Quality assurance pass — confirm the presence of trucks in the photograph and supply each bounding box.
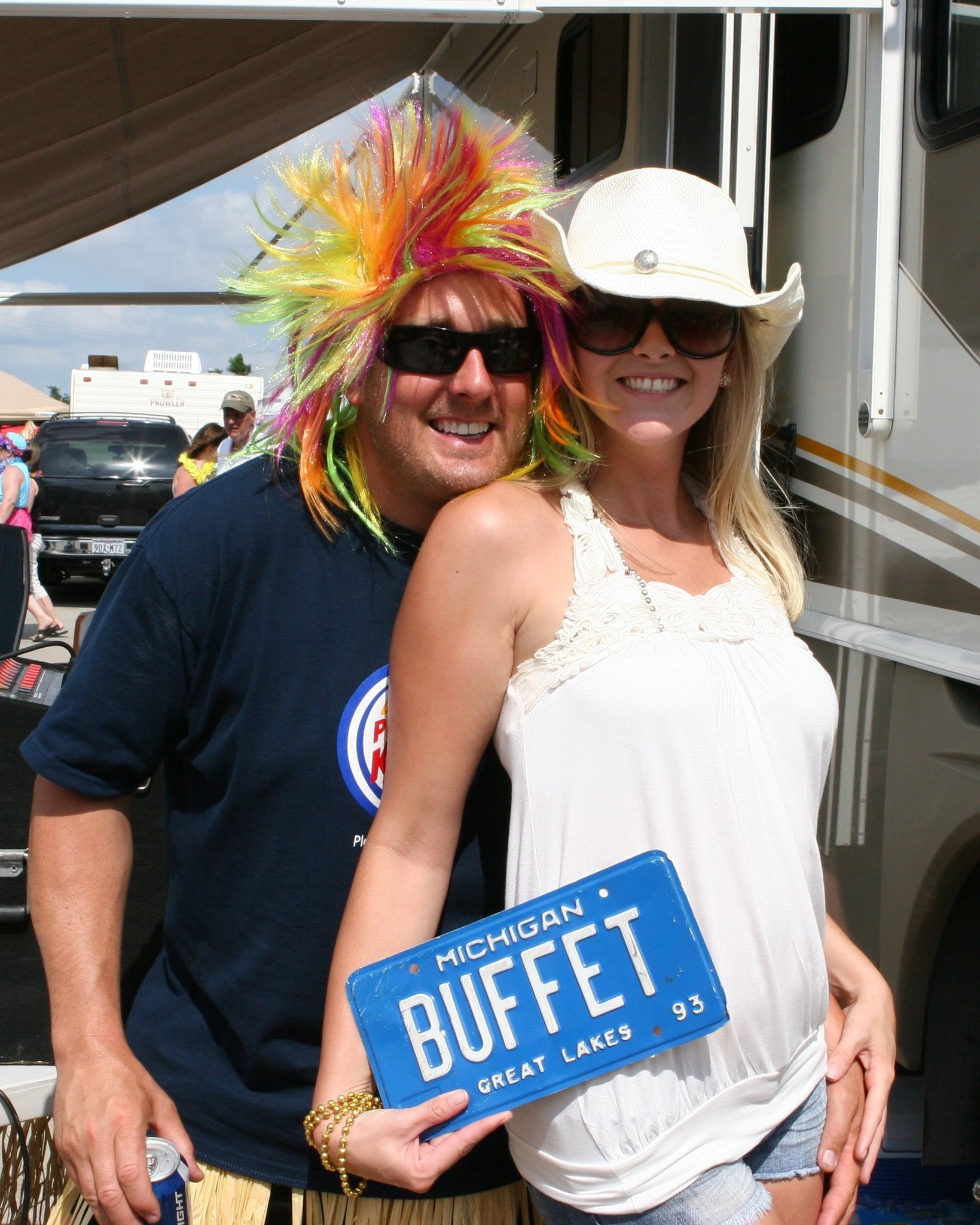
[70,366,263,446]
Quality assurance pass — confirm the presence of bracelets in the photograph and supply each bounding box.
[304,1091,384,1200]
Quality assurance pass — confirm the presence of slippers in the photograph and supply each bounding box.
[32,624,68,642]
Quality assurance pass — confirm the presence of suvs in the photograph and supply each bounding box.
[29,412,193,597]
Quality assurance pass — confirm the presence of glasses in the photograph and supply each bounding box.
[375,323,543,377]
[563,290,743,360]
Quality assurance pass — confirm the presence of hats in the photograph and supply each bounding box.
[218,390,255,413]
[529,166,806,369]
[0,432,27,457]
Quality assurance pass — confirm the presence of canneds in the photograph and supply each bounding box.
[138,1137,190,1225]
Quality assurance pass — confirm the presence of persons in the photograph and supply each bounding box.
[17,105,872,1225]
[312,169,901,1225]
[0,390,275,643]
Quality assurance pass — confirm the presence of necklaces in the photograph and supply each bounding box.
[30,470,43,479]
[179,450,214,484]
[0,456,23,474]
